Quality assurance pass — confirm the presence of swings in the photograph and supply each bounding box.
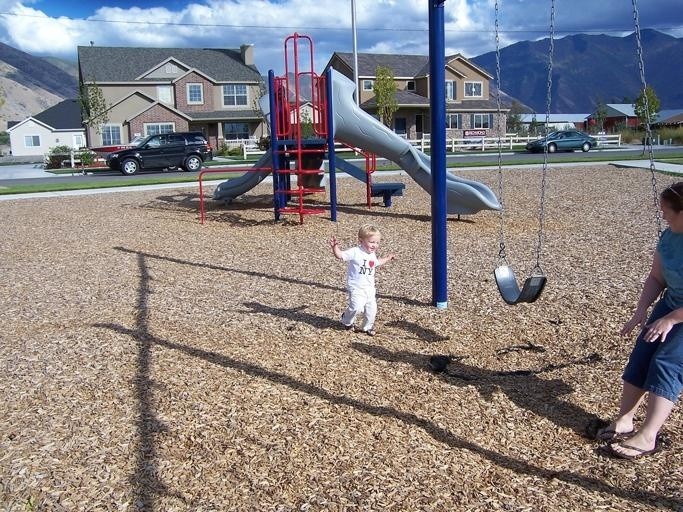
[494,0,555,306]
[630,1,683,309]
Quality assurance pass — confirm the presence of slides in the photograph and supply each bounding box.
[212,151,274,206]
[331,68,503,215]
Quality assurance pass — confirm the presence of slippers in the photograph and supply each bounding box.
[610,439,662,460]
[595,424,636,445]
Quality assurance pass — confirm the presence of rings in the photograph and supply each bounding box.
[658,331,661,335]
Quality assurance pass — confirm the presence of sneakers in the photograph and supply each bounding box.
[344,323,360,333]
[362,329,377,337]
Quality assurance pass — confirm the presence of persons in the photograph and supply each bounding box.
[596,182,683,459]
[330,224,396,335]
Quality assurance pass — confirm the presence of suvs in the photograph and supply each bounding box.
[105,131,213,177]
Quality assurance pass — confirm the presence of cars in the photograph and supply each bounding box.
[525,130,593,154]
